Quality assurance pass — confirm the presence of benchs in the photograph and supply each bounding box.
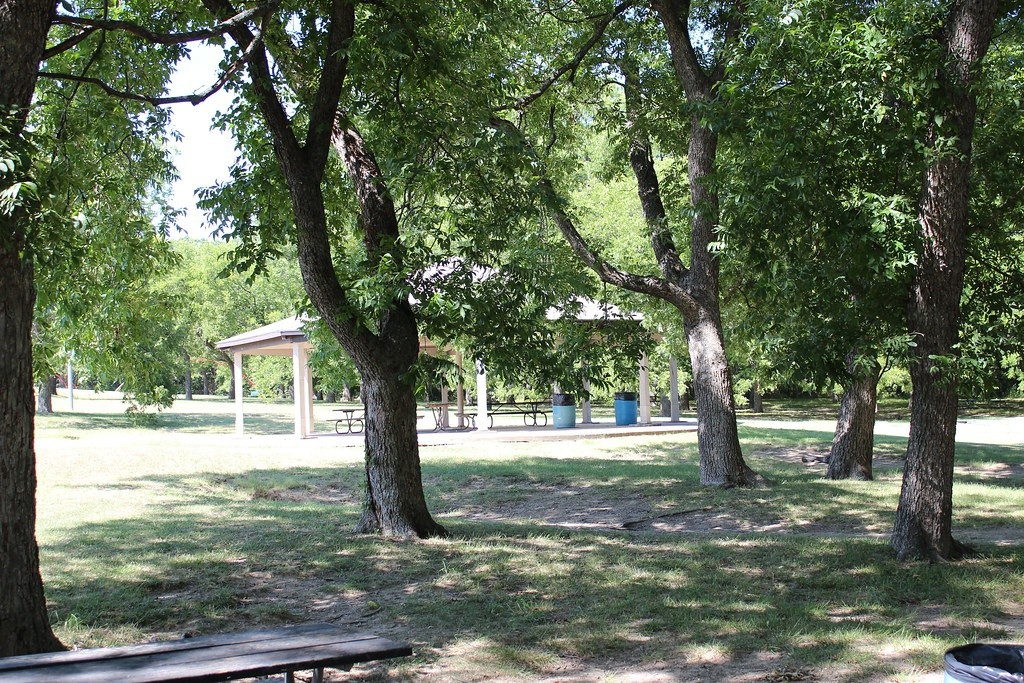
[454,410,554,432]
[327,417,365,422]
[0,624,413,683]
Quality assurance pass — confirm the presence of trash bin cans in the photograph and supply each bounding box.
[943,642,1024,683]
[553,394,576,428]
[615,392,637,426]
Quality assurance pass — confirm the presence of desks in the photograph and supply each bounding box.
[487,401,552,428]
[426,403,449,432]
[332,408,364,434]
